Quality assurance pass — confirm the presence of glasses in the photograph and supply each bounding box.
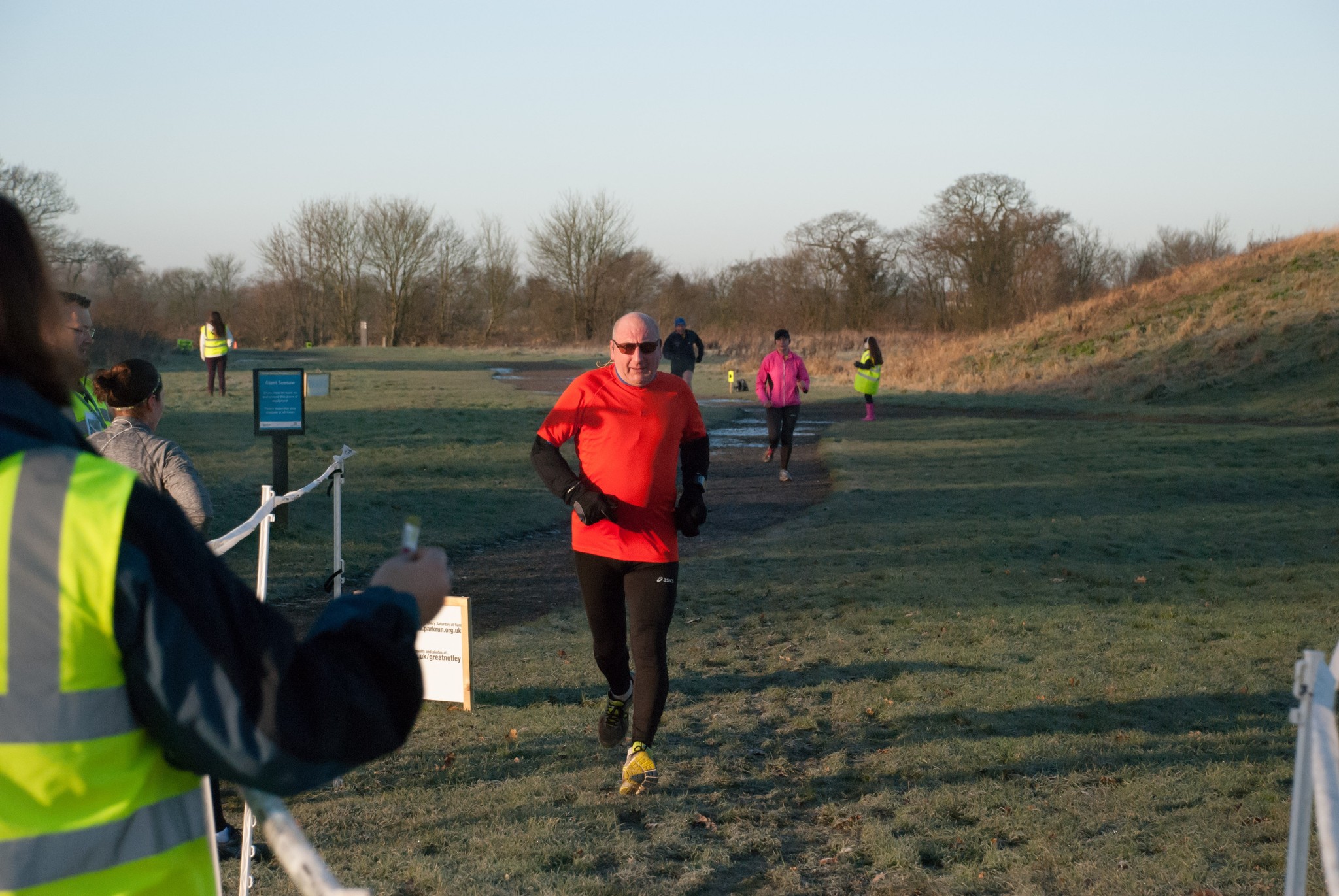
[611,339,658,355]
[58,324,96,339]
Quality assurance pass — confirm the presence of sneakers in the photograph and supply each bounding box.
[618,742,658,797]
[597,671,638,747]
[779,469,792,482]
[763,446,775,463]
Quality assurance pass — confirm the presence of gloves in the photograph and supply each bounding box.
[678,487,707,527]
[565,485,617,526]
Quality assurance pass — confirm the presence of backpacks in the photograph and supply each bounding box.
[735,379,749,392]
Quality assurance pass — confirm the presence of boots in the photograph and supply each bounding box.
[864,403,875,422]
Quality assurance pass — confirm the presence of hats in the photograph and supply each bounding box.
[674,318,686,328]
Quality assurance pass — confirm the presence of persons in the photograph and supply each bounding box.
[0,194,450,896]
[199,310,235,397]
[660,318,703,393]
[43,288,117,438]
[755,329,810,482]
[78,358,214,541]
[531,310,711,798]
[853,335,884,421]
